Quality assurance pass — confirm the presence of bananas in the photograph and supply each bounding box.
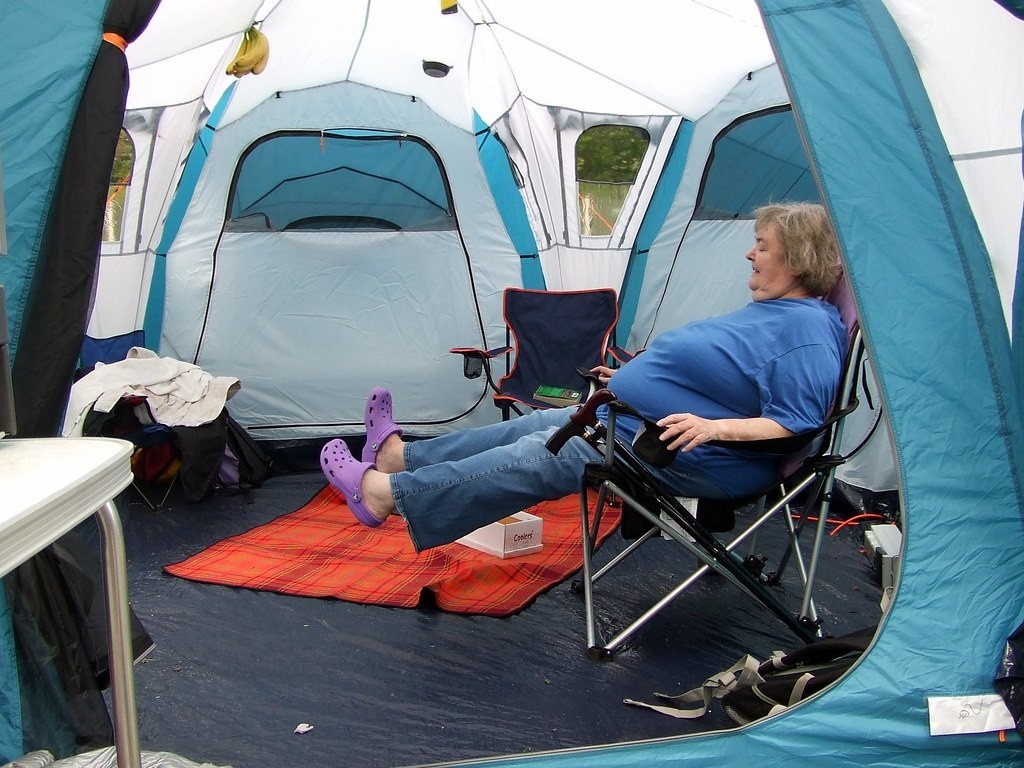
[225,26,269,78]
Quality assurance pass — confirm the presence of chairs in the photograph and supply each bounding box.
[81,331,179,511]
[450,286,632,422]
[545,267,886,664]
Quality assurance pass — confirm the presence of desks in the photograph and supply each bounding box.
[0,436,143,768]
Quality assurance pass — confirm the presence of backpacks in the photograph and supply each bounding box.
[623,624,879,727]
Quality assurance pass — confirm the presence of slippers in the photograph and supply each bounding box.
[361,389,403,464]
[320,439,387,527]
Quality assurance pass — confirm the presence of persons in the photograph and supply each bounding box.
[320,201,848,554]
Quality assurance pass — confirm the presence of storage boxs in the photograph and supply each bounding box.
[454,511,542,558]
